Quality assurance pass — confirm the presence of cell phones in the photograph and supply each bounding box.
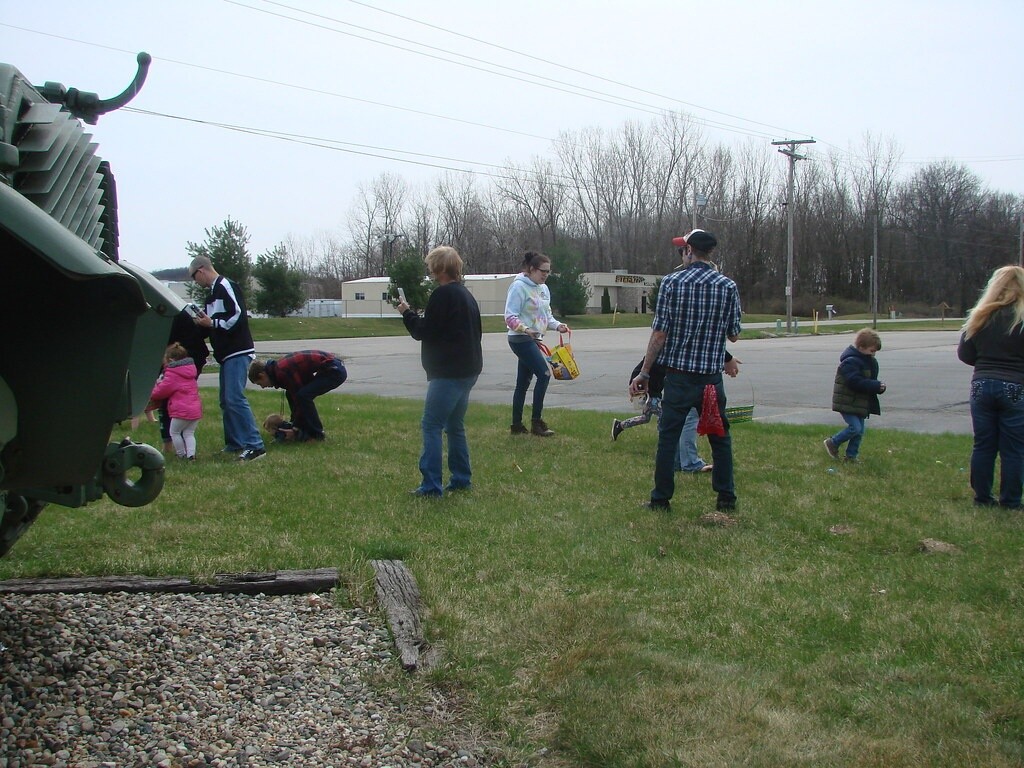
[398,287,409,305]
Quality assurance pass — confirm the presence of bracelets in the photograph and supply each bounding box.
[640,372,650,378]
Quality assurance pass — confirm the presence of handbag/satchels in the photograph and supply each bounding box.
[539,328,580,381]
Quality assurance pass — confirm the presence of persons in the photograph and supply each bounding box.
[504,251,568,437]
[398,246,484,497]
[957,265,1024,512]
[629,229,741,513]
[823,329,886,465]
[159,309,210,453]
[188,256,266,464]
[612,355,666,442]
[151,342,202,461]
[674,406,713,474]
[249,349,347,443]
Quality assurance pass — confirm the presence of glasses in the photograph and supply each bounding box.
[190,266,202,281]
[536,268,552,276]
[678,246,689,255]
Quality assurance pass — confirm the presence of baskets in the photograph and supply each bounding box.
[723,370,756,423]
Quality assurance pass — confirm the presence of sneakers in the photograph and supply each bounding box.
[511,422,532,433]
[531,420,555,436]
[237,449,265,461]
[221,445,237,452]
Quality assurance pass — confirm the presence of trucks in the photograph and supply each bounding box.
[0,46,181,559]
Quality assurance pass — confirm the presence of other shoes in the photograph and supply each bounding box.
[176,454,187,462]
[824,438,839,458]
[842,456,861,464]
[188,455,196,462]
[445,484,470,493]
[408,489,423,496]
[611,418,624,441]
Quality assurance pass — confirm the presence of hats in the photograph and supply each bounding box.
[688,232,717,252]
[672,228,705,247]
